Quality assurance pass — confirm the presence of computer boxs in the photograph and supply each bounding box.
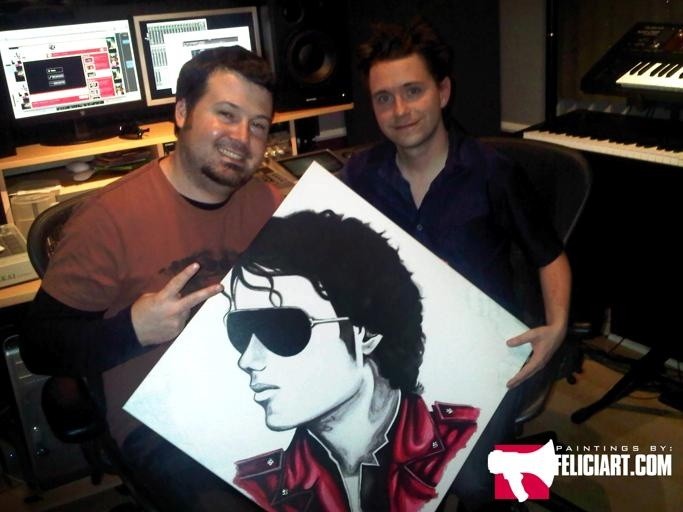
[3,335,106,489]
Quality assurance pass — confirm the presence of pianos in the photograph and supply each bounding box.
[581,21,683,102]
[512,109,683,168]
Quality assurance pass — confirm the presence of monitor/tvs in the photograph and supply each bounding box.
[132,6,264,109]
[0,16,148,147]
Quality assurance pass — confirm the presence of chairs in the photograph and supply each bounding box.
[26,181,186,510]
[466,128,598,511]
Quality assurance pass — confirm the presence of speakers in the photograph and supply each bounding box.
[257,0,353,108]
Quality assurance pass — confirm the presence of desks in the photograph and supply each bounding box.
[0,95,393,311]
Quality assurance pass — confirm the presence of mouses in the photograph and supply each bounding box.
[66,161,90,173]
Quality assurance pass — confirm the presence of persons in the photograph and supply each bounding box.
[218,208,482,512]
[344,14,575,512]
[19,45,287,511]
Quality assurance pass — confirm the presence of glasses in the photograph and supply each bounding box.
[226,307,349,357]
